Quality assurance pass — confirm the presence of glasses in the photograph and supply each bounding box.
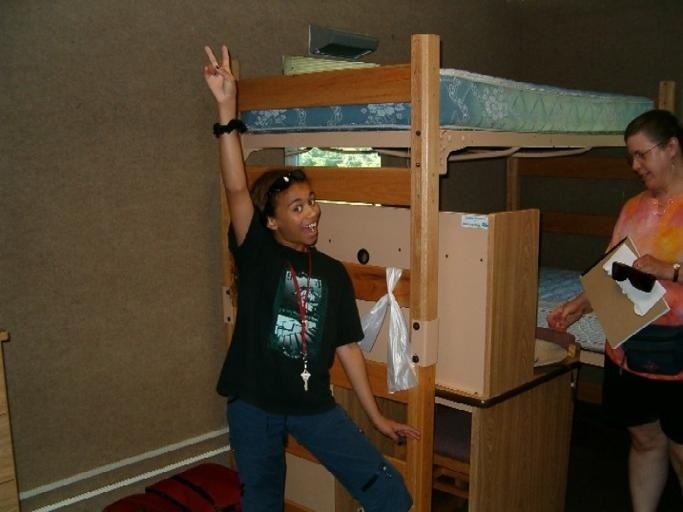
[623,128,682,170]
[610,259,657,294]
[267,168,305,194]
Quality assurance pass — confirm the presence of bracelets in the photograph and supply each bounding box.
[212,119,248,138]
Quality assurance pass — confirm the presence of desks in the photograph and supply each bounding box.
[313,203,583,512]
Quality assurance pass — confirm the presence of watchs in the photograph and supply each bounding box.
[672,263,681,282]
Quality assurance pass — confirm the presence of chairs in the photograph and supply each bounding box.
[453,328,577,511]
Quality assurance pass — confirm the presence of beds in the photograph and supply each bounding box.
[214,33,678,512]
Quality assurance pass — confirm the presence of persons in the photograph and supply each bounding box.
[547,109,682,512]
[202,45,422,512]
